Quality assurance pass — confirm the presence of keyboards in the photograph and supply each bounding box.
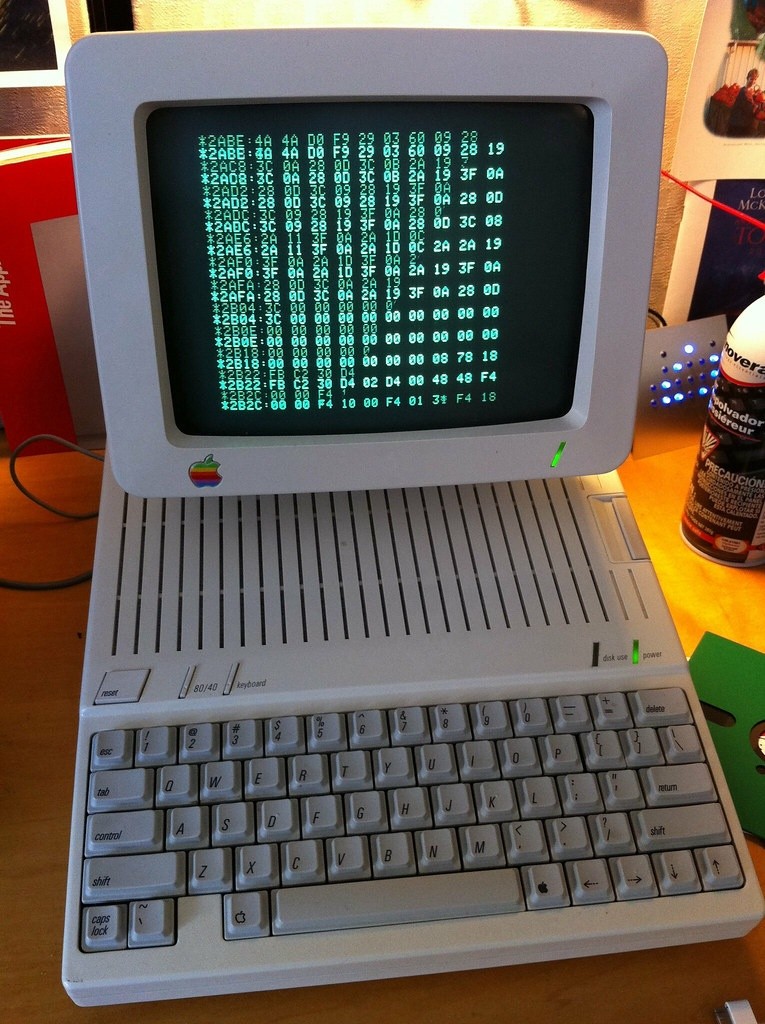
[77,687,746,952]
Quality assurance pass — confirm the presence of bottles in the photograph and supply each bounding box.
[680,296,765,570]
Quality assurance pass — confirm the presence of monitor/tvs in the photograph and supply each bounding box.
[0,0,73,88]
[72,26,669,498]
